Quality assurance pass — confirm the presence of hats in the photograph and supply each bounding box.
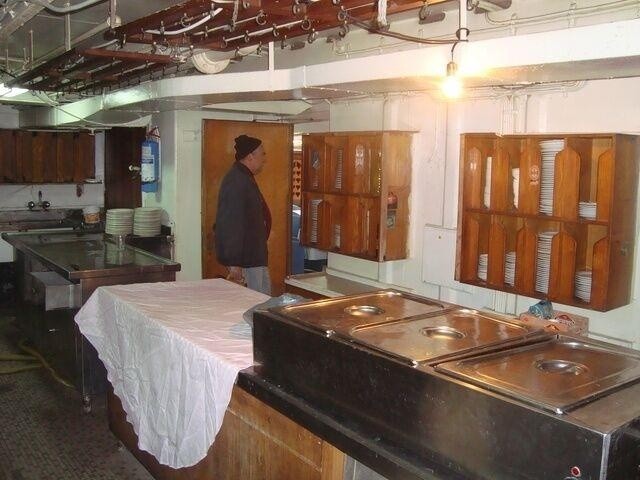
[232,132,263,162]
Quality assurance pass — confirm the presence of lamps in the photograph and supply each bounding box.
[439,60,465,101]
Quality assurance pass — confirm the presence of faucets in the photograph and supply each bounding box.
[39,190,42,203]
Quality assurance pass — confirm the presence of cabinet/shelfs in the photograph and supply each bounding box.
[299,130,421,264]
[453,133,640,312]
[0,127,96,184]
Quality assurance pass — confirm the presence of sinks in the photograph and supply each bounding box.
[16,223,74,231]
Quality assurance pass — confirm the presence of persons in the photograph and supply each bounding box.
[213,133,274,297]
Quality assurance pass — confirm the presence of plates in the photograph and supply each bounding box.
[309,147,345,248]
[105,208,163,236]
[477,141,597,303]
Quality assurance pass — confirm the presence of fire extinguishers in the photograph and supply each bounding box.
[141,126,161,193]
